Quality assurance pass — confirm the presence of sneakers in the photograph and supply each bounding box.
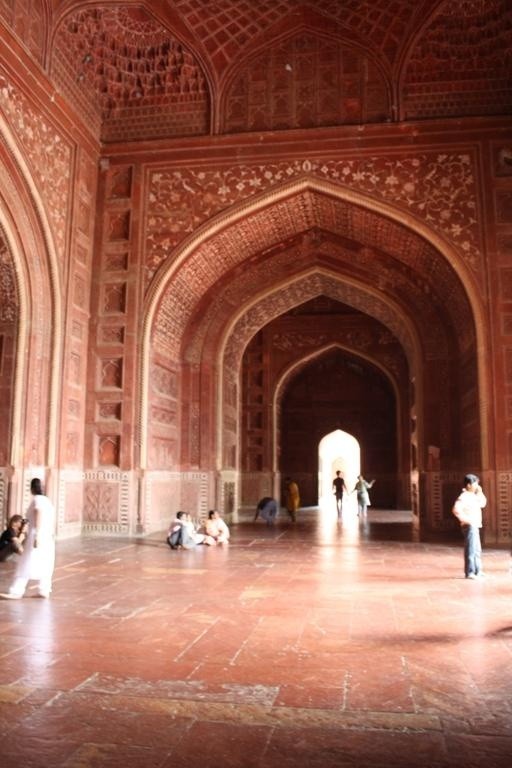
[0,592,22,599]
[467,572,488,579]
[38,592,50,599]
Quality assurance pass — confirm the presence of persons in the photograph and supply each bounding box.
[330,470,377,519]
[251,476,301,528]
[0,478,57,600]
[166,510,231,551]
[451,471,488,578]
